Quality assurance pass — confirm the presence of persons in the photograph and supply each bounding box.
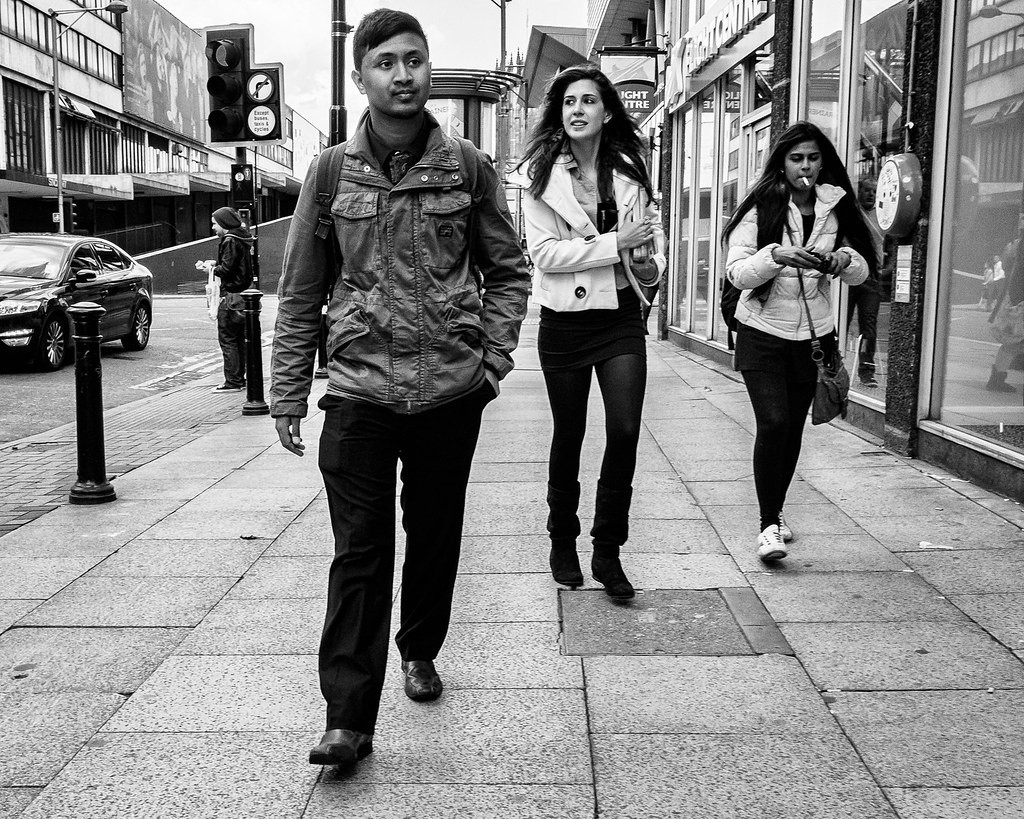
[724,121,877,559]
[270,9,529,764]
[203,207,254,392]
[127,8,205,138]
[514,65,665,600]
[979,230,1024,392]
[847,179,896,386]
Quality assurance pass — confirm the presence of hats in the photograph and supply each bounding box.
[213,207,242,231]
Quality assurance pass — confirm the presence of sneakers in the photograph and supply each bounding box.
[757,524,788,559]
[778,511,794,541]
[859,371,878,387]
[211,382,246,393]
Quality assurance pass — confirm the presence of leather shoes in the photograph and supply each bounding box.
[309,725,374,764]
[402,652,442,701]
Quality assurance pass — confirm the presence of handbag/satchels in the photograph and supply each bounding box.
[990,292,1024,346]
[812,364,849,425]
[206,269,219,321]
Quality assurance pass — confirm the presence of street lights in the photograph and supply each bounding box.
[48,2,129,231]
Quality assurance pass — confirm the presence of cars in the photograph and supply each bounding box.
[0,233,154,372]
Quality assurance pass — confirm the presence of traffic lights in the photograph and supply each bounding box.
[204,24,254,147]
[63,198,77,232]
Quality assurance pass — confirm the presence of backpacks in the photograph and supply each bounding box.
[721,199,846,348]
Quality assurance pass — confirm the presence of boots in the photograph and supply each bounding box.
[986,366,1015,391]
[591,479,635,599]
[547,480,584,586]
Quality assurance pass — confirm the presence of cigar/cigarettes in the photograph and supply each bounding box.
[802,177,811,188]
